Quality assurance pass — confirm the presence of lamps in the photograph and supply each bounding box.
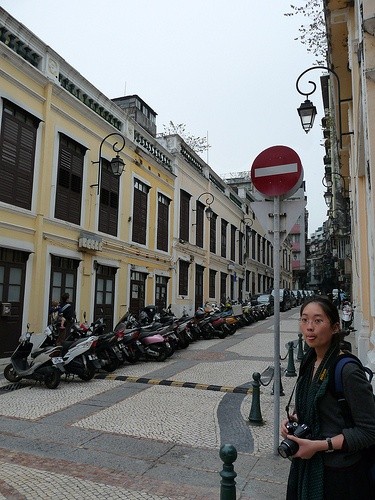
[236,218,253,242]
[295,66,354,148]
[90,133,126,195]
[192,192,214,226]
[321,171,352,235]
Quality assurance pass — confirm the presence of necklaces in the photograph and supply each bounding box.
[314,362,319,368]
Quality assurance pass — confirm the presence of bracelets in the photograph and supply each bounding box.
[325,438,334,453]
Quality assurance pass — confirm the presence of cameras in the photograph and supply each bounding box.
[277,421,312,458]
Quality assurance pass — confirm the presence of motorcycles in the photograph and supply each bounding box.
[27,287,317,391]
[3,321,66,390]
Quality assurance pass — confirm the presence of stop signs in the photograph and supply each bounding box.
[251,145,302,196]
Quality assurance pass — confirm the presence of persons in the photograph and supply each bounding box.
[49,292,76,337]
[281,294,375,500]
